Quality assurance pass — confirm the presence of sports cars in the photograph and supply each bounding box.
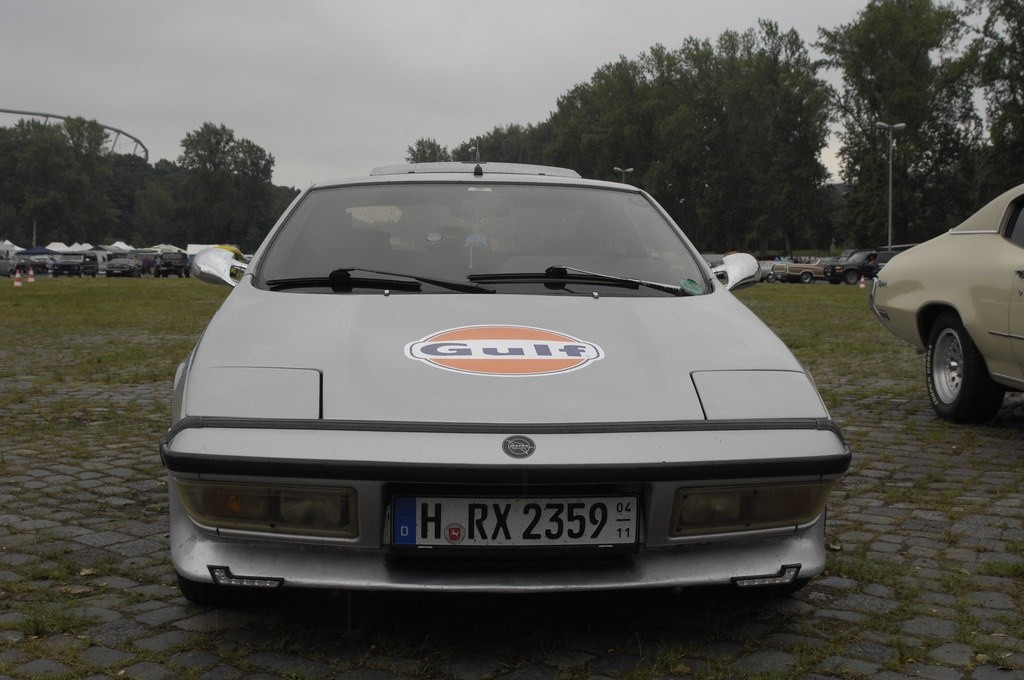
[871,182,1023,425]
[159,161,854,631]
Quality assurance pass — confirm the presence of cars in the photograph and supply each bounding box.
[694,242,926,288]
[0,238,258,279]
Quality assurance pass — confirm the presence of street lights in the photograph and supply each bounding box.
[875,122,908,254]
[612,165,636,185]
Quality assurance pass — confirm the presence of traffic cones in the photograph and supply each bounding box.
[13,268,23,288]
[27,265,36,283]
[858,274,867,287]
